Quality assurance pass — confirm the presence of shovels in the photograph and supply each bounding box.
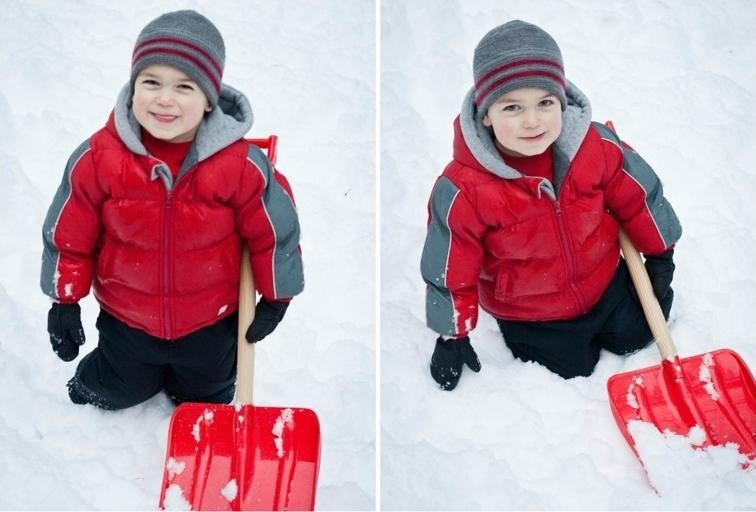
[606,121,756,498]
[160,136,322,511]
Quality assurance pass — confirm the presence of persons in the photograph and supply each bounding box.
[417,16,686,395]
[36,8,307,409]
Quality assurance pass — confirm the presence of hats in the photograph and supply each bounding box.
[130,10,226,110]
[473,20,568,120]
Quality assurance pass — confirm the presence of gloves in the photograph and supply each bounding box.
[228,294,292,344]
[429,336,481,391]
[642,252,678,304]
[45,298,87,364]
